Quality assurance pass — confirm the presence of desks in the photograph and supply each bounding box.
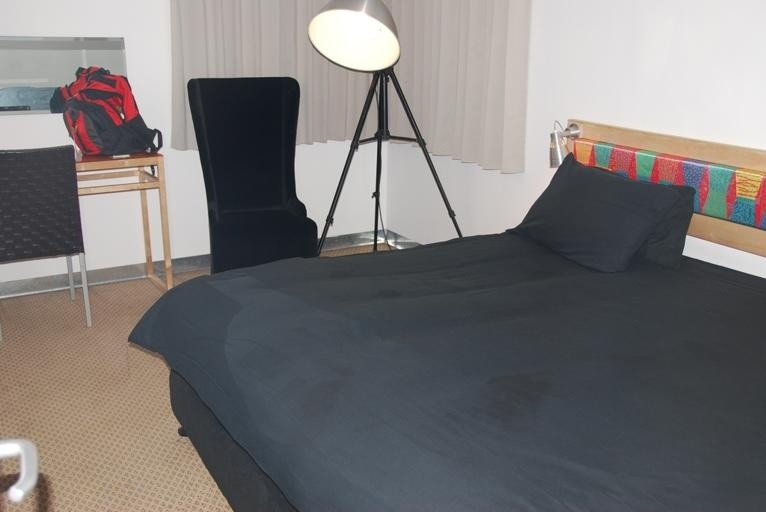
[76,152,173,293]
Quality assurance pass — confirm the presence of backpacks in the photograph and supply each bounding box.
[49,66,163,154]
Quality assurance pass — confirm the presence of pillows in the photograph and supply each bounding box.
[505,151,680,273]
[636,185,695,272]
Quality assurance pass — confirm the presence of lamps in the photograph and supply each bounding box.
[308,0,464,255]
[549,120,580,168]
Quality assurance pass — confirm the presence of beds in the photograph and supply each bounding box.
[129,119,766,512]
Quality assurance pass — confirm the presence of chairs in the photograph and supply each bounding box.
[186,76,319,277]
[0,145,92,343]
